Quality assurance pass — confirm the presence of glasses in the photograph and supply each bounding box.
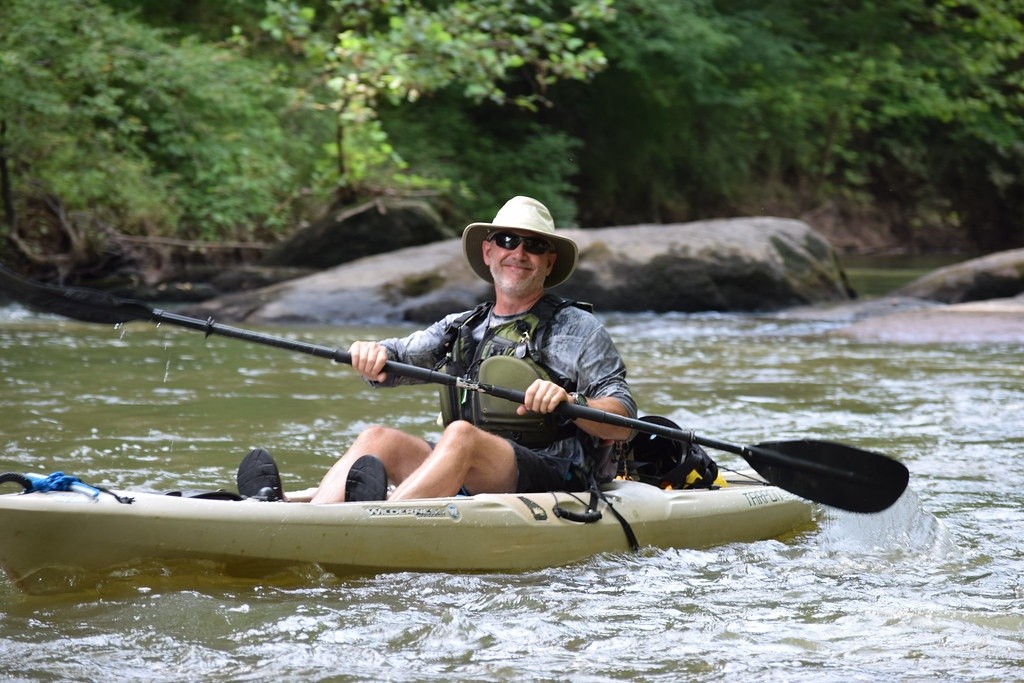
[488,232,553,255]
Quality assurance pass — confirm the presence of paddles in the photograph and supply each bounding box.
[29,267,909,515]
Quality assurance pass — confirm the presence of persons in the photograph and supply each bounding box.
[235,195,637,504]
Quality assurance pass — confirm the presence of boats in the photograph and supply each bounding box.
[1,470,827,596]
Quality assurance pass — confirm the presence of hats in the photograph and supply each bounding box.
[461,196,579,291]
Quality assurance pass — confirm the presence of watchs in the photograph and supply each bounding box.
[567,391,588,405]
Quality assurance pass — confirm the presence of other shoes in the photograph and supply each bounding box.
[237,450,284,503]
[344,455,387,502]
